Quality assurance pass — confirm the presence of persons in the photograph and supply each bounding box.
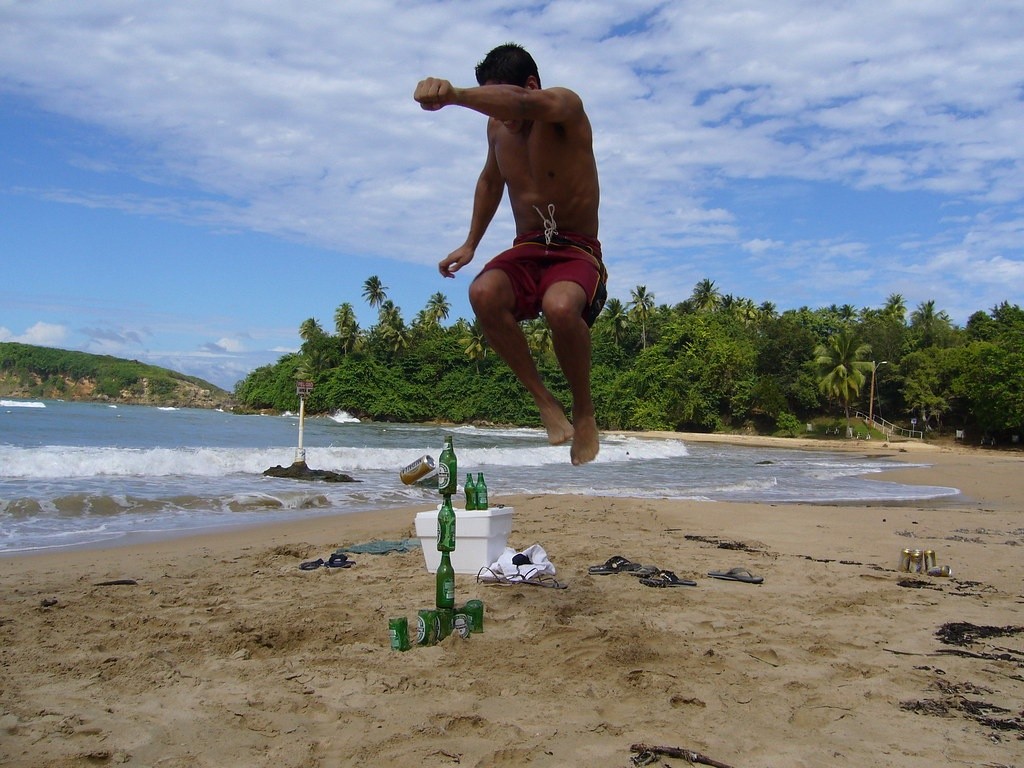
[413,40,609,466]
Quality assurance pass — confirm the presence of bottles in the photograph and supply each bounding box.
[435,551,454,607]
[436,494,456,551]
[475,472,488,511]
[464,472,477,510]
[437,435,457,494]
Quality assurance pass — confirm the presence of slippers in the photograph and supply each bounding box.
[515,567,568,590]
[629,564,660,578]
[589,556,642,575]
[639,568,699,587]
[707,565,764,585]
[325,553,355,568]
[297,558,323,570]
[476,566,510,589]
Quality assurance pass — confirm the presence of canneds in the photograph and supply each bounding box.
[400,455,436,486]
[388,599,483,651]
[897,546,952,577]
